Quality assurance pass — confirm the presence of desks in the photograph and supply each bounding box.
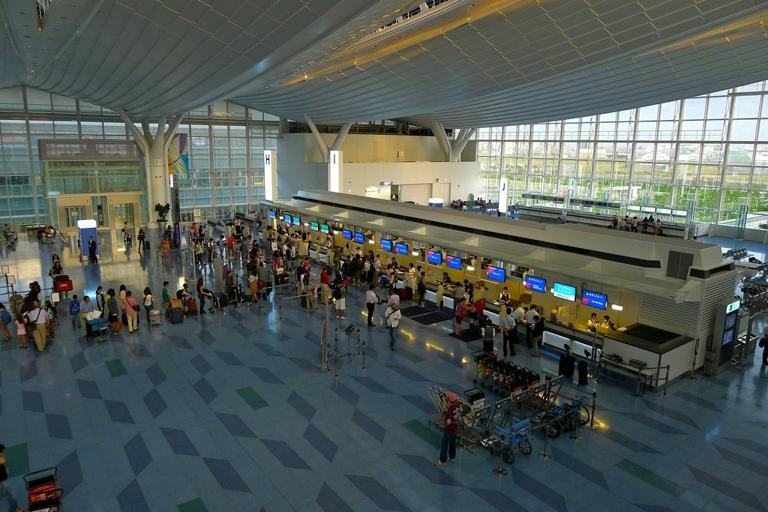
[600,356,653,396]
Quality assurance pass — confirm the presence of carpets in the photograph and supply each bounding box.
[399,304,482,343]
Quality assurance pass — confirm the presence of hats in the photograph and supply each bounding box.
[447,391,457,402]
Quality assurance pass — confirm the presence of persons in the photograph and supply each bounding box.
[453,197,491,211]
[602,314,615,331]
[588,312,600,333]
[610,215,662,236]
[762,326,768,365]
[434,393,463,465]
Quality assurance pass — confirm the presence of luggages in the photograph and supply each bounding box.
[301,289,312,308]
[53,274,73,292]
[149,309,160,326]
[162,298,198,324]
[122,311,129,326]
[204,283,246,313]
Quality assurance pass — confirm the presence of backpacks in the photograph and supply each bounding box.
[333,285,343,301]
[176,290,183,300]
[49,307,57,320]
[0,308,11,325]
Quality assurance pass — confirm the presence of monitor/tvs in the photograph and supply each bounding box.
[525,275,546,293]
[581,289,608,310]
[553,282,576,302]
[269,207,462,271]
[487,266,506,283]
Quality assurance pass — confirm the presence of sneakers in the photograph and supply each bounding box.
[435,460,447,466]
[336,315,346,319]
[448,457,454,462]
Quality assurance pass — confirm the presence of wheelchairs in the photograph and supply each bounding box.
[483,394,590,464]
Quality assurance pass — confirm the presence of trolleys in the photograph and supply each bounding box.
[205,283,253,315]
[83,311,110,343]
[425,349,563,443]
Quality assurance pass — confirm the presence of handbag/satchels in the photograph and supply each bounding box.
[114,321,122,331]
[71,304,79,315]
[24,322,36,332]
[133,304,141,312]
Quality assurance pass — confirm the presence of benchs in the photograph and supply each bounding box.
[2,224,61,247]
